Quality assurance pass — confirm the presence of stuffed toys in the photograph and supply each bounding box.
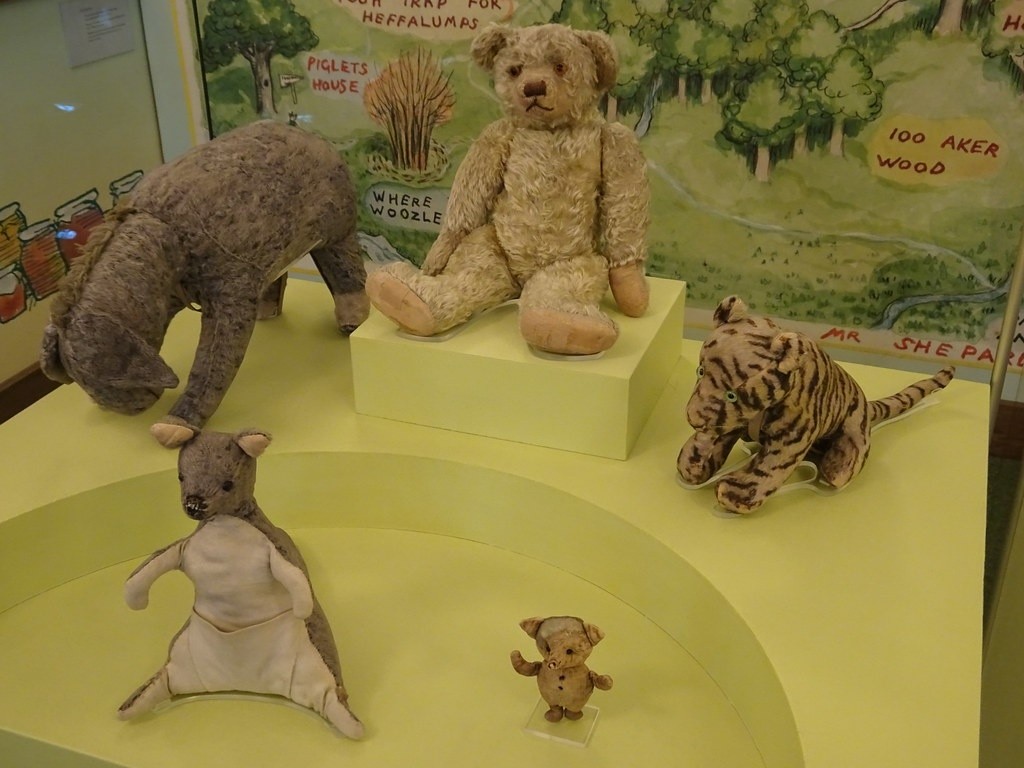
[678,295,953,513]
[513,616,614,721]
[114,422,367,742]
[366,24,649,355]
[42,124,370,446]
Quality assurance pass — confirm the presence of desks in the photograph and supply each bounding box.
[0,280,988,768]
